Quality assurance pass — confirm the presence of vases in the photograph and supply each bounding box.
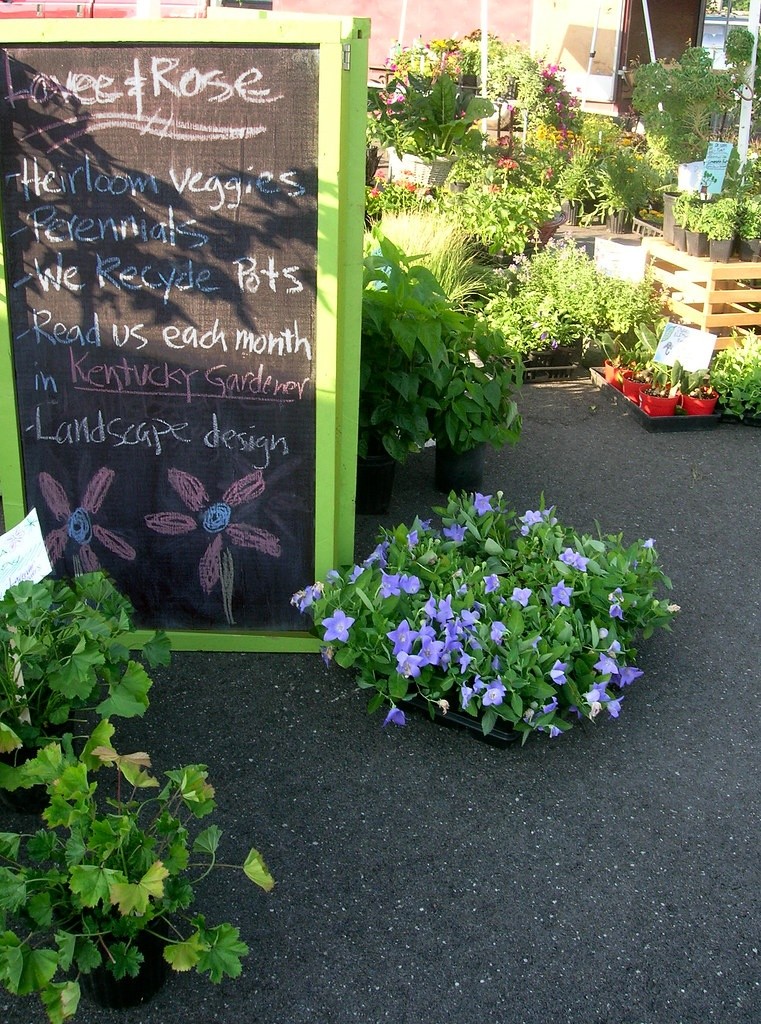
[392,674,523,747]
[528,351,554,379]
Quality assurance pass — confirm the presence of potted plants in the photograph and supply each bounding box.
[583,308,761,426]
[362,74,761,264]
[0,577,275,1024]
[356,225,526,512]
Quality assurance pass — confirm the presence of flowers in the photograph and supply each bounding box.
[527,327,560,353]
[293,486,685,741]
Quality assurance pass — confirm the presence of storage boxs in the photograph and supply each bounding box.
[590,367,721,434]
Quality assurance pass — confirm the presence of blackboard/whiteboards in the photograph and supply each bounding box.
[0,16,372,653]
